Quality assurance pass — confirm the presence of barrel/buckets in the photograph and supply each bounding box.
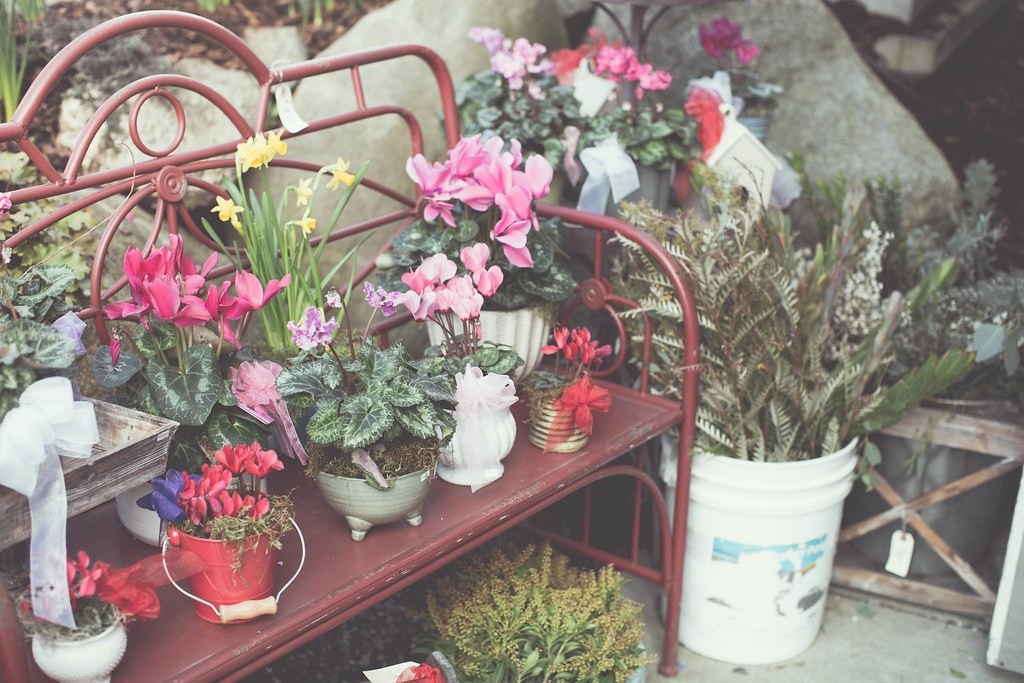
[650,396,865,667]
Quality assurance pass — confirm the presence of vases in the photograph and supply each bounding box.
[313,468,433,542]
[29,618,128,683]
[734,115,770,145]
[162,517,306,625]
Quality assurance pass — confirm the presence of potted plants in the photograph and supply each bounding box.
[614,154,1023,666]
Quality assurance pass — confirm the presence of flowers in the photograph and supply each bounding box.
[697,14,785,120]
[0,114,636,493]
[141,431,297,590]
[10,544,164,641]
[0,7,703,683]
